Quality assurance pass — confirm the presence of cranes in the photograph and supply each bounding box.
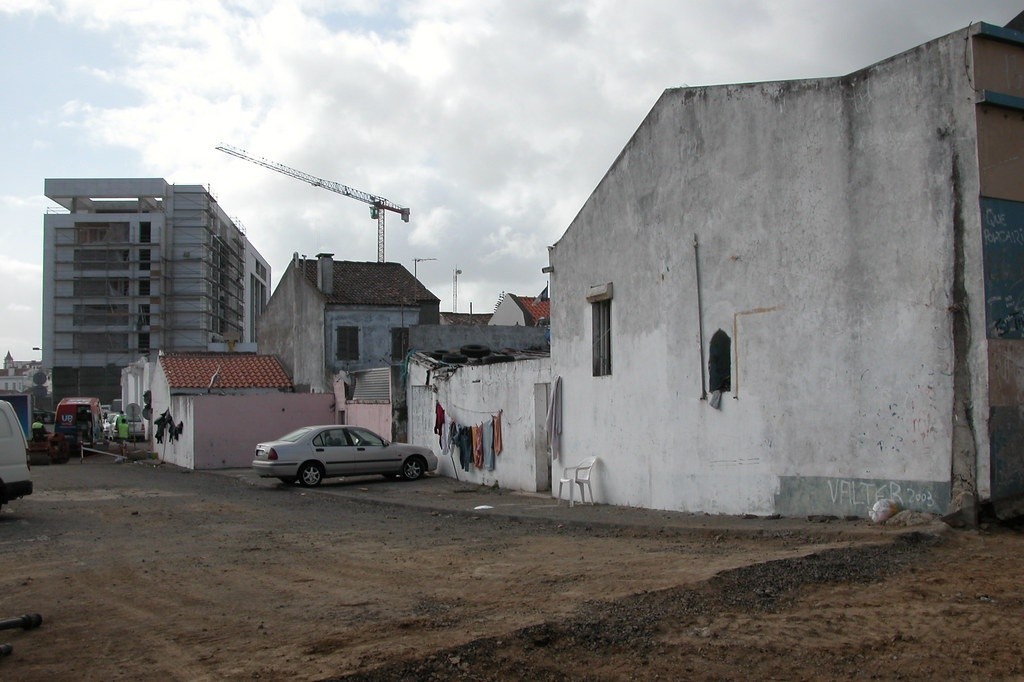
[211,140,410,262]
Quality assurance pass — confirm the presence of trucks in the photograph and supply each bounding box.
[53,396,100,447]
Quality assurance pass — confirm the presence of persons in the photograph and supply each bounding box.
[117,419,128,443]
[32,417,51,442]
[115,411,126,430]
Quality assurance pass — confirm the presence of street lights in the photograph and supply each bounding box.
[31,346,85,397]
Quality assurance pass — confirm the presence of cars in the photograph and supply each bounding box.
[0,398,35,510]
[252,426,439,489]
[100,399,145,440]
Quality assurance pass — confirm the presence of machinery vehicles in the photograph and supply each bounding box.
[451,265,462,312]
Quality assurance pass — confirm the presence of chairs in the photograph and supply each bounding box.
[558,455,598,508]
